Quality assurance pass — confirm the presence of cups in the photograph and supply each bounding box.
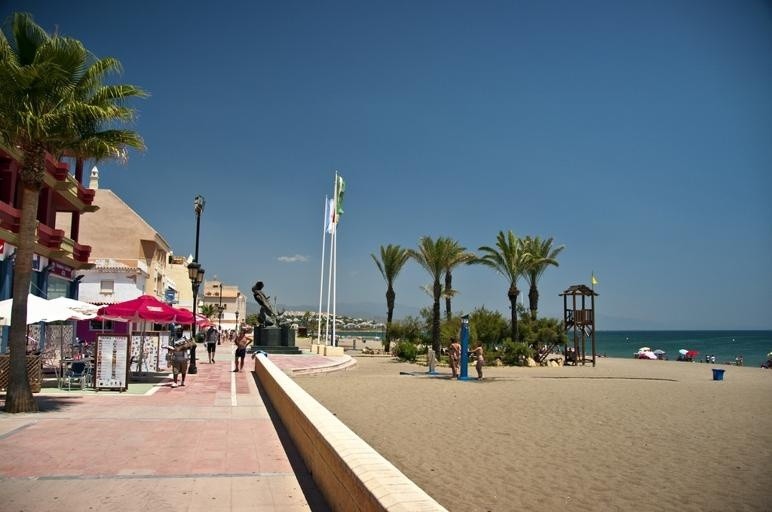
[81,353,85,359]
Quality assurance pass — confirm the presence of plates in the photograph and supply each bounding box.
[64,358,75,361]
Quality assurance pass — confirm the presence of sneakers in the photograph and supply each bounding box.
[171,381,184,387]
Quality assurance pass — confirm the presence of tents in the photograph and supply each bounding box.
[639,352,657,359]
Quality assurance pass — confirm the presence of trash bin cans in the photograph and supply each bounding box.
[197,333,205,343]
[712,369,725,380]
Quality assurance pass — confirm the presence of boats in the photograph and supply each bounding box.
[633,347,654,356]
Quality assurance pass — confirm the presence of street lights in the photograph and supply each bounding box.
[461,315,468,377]
[217,284,225,344]
[233,311,239,333]
[187,194,205,374]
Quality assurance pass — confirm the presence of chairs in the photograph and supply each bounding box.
[129,353,149,375]
[58,362,85,392]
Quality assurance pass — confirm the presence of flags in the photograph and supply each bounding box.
[325,174,346,234]
[592,277,599,284]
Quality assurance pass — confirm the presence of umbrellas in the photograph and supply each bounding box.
[687,350,697,356]
[654,349,665,354]
[639,346,650,351]
[679,348,688,355]
[0,294,216,389]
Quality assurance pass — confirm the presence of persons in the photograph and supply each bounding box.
[470,340,484,378]
[232,330,253,372]
[735,356,739,365]
[678,353,695,362]
[740,356,743,366]
[700,354,715,363]
[448,336,461,378]
[221,328,236,343]
